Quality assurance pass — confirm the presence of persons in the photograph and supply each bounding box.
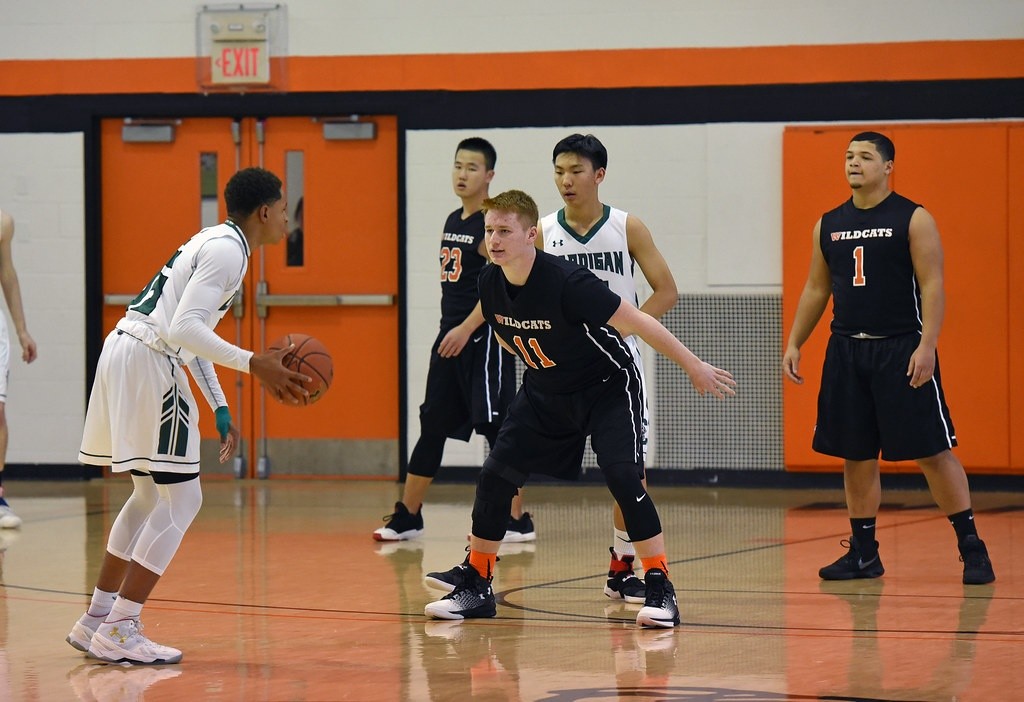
[374,539,678,702]
[780,132,997,585]
[421,191,738,630]
[1,211,37,529]
[67,663,184,702]
[819,580,996,702]
[371,135,537,544]
[286,197,303,266]
[424,130,680,602]
[61,167,312,666]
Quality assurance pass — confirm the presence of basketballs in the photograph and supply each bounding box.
[265,332,336,407]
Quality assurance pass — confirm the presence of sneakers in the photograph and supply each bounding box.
[87,618,183,665]
[958,584,995,624]
[637,625,682,669]
[957,535,996,586]
[425,552,470,591]
[425,562,497,620]
[0,487,23,529]
[636,567,680,628]
[67,659,184,700]
[372,501,425,542]
[496,543,536,571]
[820,578,885,614]
[373,540,424,572]
[65,609,109,653]
[604,601,645,630]
[424,617,497,670]
[604,547,647,605]
[467,511,537,542]
[818,536,885,580]
